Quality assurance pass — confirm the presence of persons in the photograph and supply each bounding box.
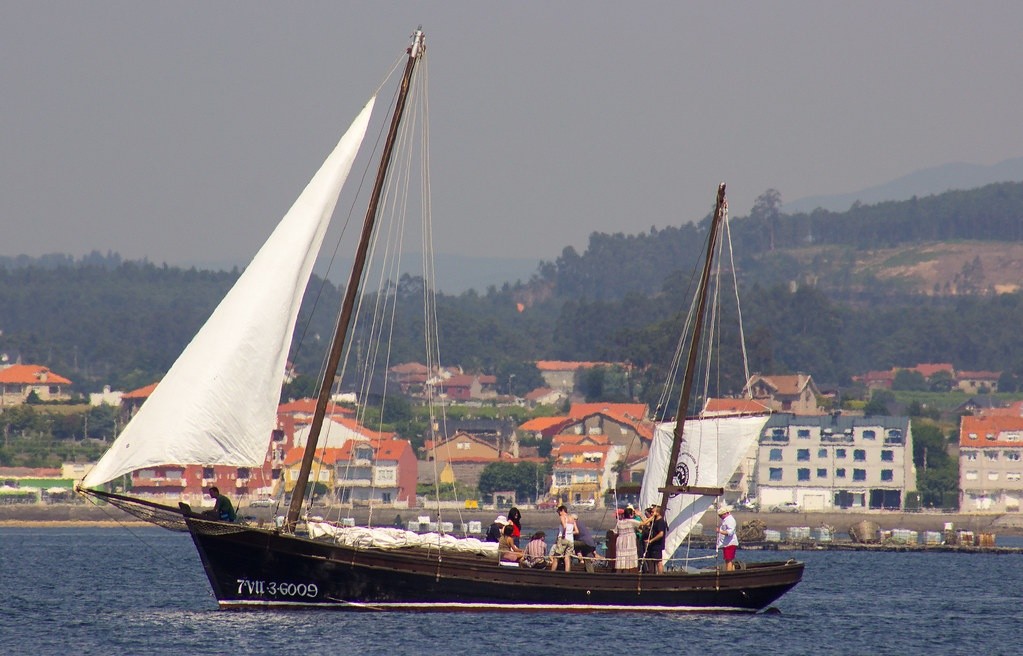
[610,506,665,574]
[486,506,601,572]
[714,507,739,571]
[201,487,235,523]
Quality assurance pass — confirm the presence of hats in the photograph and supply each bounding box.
[535,532,548,539]
[611,509,624,517]
[718,508,729,514]
[494,516,509,526]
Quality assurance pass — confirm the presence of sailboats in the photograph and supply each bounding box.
[74,22,808,616]
